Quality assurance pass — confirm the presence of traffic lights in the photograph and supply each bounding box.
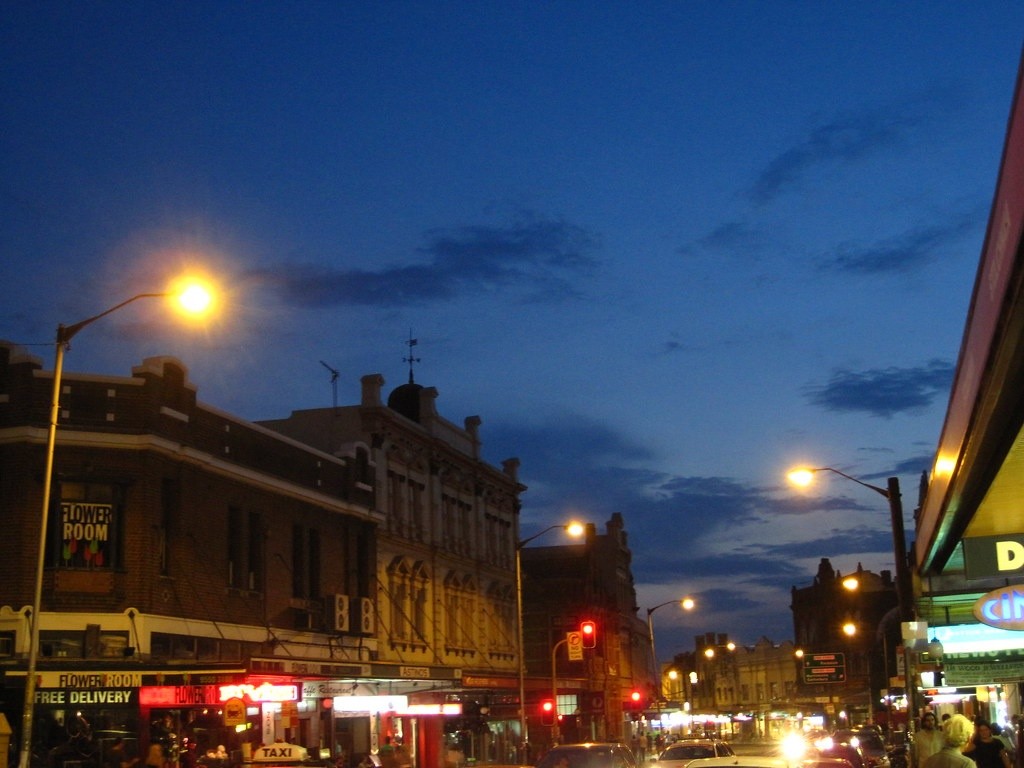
[539,700,555,727]
[631,688,643,715]
[581,621,597,650]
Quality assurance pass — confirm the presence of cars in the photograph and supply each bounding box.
[655,737,738,768]
[791,722,892,768]
[534,742,636,768]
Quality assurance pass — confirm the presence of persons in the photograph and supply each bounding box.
[380,736,395,768]
[865,718,872,725]
[557,732,618,745]
[358,756,375,768]
[525,743,531,765]
[630,727,675,762]
[888,721,907,745]
[336,758,344,768]
[914,711,1024,768]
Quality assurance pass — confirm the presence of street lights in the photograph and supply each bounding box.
[842,622,894,739]
[782,465,915,730]
[513,520,586,768]
[647,596,697,747]
[793,648,851,726]
[18,276,222,767]
